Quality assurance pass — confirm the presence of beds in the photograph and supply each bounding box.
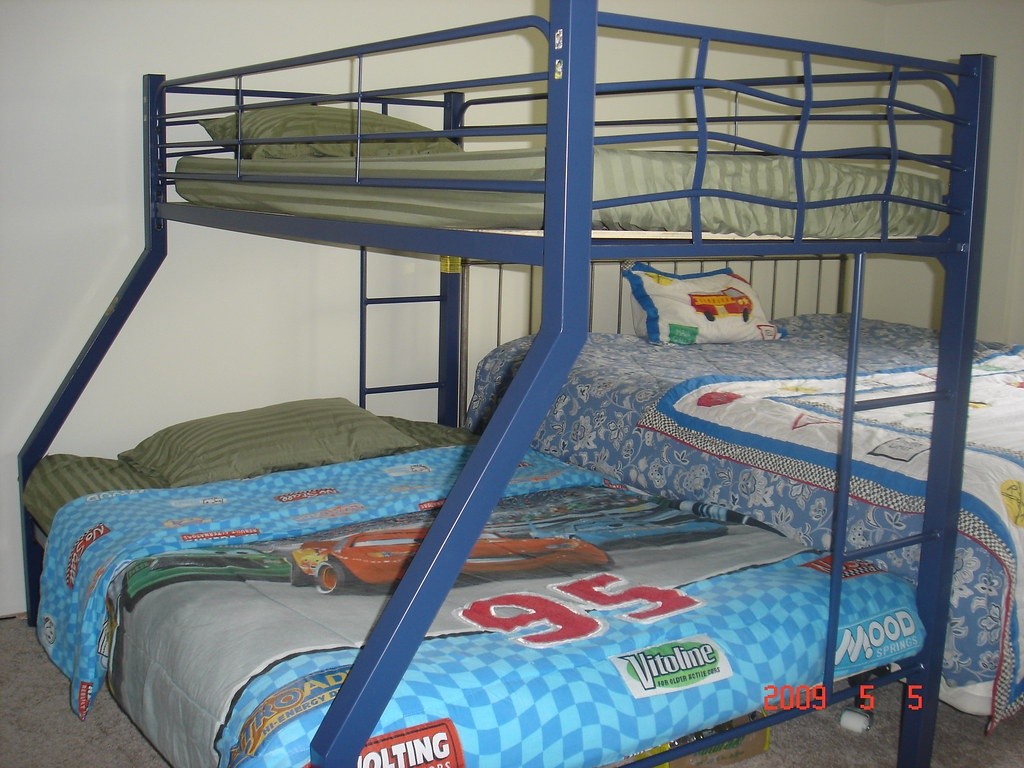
[459,258,1023,735]
[19,1,998,768]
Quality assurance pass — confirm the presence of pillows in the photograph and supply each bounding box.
[117,397,417,487]
[624,262,787,346]
[199,105,463,161]
